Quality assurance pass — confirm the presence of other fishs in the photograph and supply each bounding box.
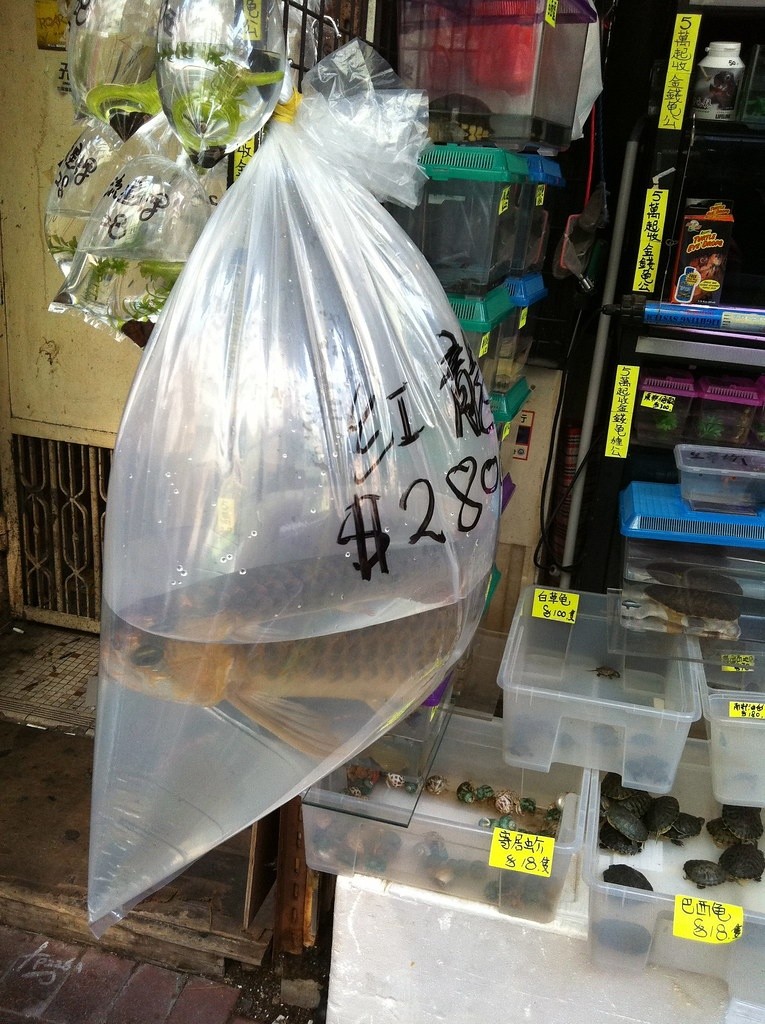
[98,487,494,765]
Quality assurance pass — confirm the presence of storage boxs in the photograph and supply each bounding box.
[298,1,765,1024]
